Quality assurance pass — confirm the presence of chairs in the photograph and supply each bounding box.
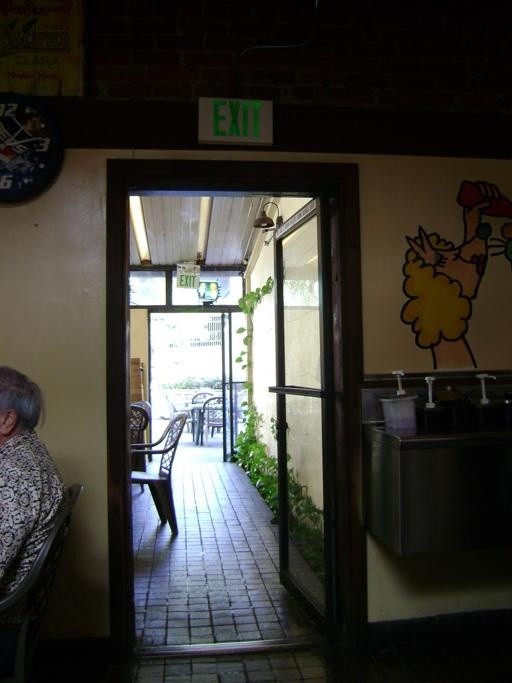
[132,391,227,538]
[0,483,85,683]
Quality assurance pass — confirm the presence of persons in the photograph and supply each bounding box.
[0,366,69,625]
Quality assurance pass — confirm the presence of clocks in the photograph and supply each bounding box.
[0,91,69,210]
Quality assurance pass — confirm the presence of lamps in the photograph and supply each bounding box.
[198,278,220,305]
[252,199,283,244]
